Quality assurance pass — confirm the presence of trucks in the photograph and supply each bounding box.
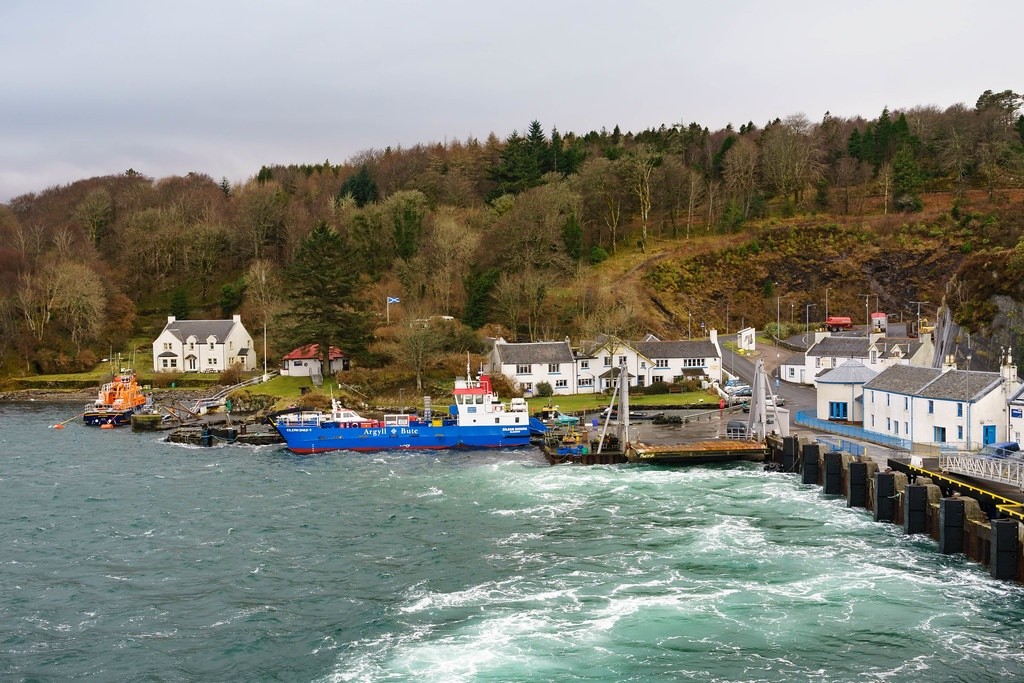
[820,317,853,332]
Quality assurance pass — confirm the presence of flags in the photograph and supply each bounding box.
[389,297,400,303]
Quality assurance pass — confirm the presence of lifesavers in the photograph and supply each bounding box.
[352,422,359,428]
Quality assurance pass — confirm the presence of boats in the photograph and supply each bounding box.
[601,406,665,419]
[267,350,531,454]
[194,402,226,416]
[529,393,584,425]
[47,344,161,428]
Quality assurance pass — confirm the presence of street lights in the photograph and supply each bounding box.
[909,301,930,338]
[778,297,780,340]
[807,304,817,347]
[792,302,794,324]
[826,288,833,321]
[858,294,869,338]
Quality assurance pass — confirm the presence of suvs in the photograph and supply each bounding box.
[973,441,1020,463]
[726,420,770,438]
[729,387,752,396]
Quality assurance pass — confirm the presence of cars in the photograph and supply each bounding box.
[766,394,785,407]
[1001,450,1023,464]
[724,380,750,393]
[760,414,774,423]
[743,398,757,412]
